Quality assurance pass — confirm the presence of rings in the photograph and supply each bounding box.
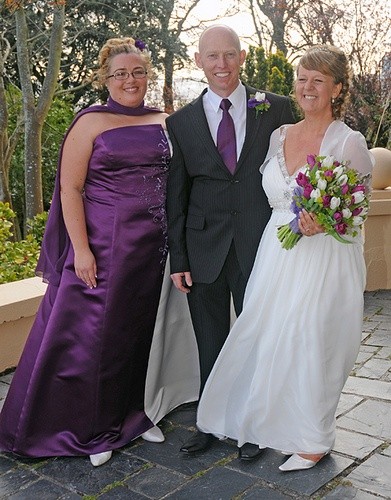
[306,228,310,232]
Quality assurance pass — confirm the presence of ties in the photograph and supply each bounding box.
[217,98,237,175]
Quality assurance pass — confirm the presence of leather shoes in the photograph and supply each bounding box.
[277,446,336,472]
[88,449,113,466]
[180,430,215,456]
[281,451,293,456]
[142,426,165,443]
[239,443,264,461]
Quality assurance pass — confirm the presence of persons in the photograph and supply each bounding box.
[165,24,295,460]
[0,36,237,466]
[194,44,375,471]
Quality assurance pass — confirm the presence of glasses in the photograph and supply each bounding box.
[105,69,148,80]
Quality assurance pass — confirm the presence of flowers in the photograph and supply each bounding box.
[275,152,374,251]
[247,91,272,119]
